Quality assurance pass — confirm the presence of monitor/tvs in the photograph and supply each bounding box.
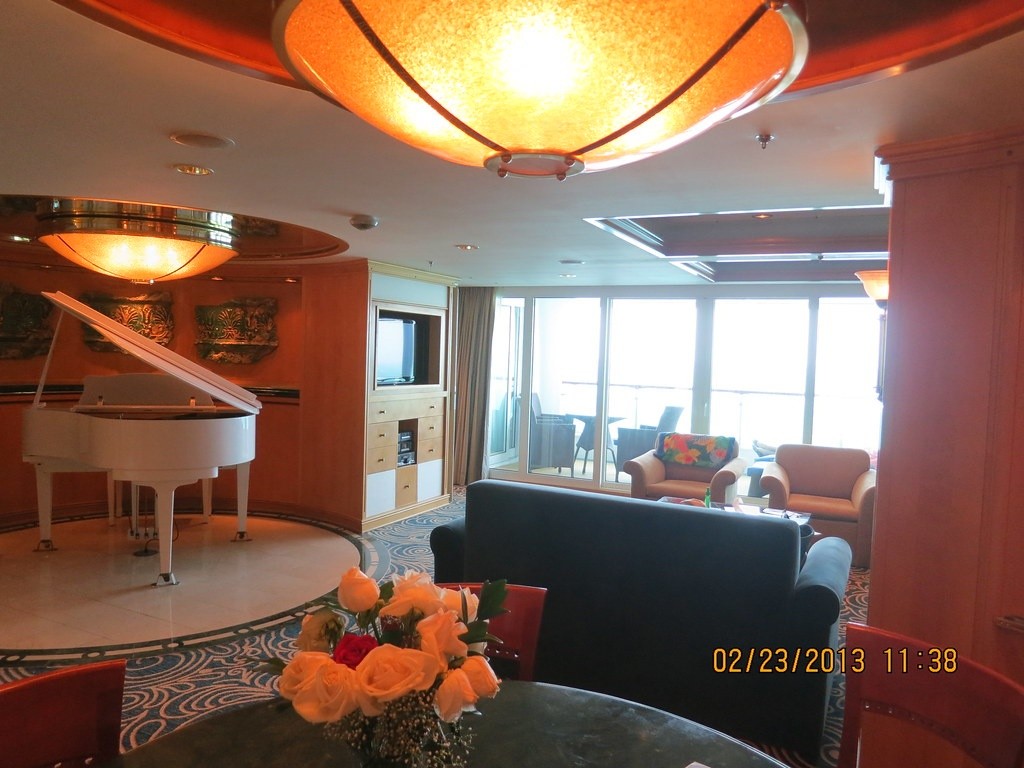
[376,315,429,386]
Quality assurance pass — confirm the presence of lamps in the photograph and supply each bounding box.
[276,0,814,182]
[38,199,240,286]
[855,265,888,306]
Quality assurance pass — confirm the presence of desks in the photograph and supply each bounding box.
[567,412,625,475]
[91,676,793,768]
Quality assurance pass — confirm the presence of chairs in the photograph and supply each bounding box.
[613,404,685,483]
[516,388,577,474]
[0,655,129,768]
[838,622,1024,768]
[422,578,548,680]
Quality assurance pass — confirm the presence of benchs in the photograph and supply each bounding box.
[108,473,213,528]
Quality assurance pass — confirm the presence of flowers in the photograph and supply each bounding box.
[268,568,507,768]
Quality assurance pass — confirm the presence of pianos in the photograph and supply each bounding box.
[19,289,264,589]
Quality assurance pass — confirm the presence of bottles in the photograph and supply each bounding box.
[705,487,712,508]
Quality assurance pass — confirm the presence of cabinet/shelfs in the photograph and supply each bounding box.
[366,389,446,531]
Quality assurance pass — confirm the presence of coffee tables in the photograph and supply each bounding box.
[656,496,811,528]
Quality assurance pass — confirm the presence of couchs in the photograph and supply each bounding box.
[622,431,747,508]
[759,444,877,567]
[428,480,854,757]
